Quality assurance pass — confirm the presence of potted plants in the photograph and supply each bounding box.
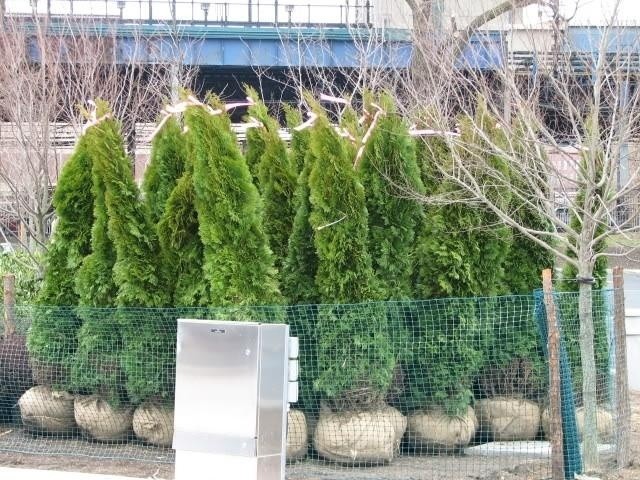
[18,83,611,466]
